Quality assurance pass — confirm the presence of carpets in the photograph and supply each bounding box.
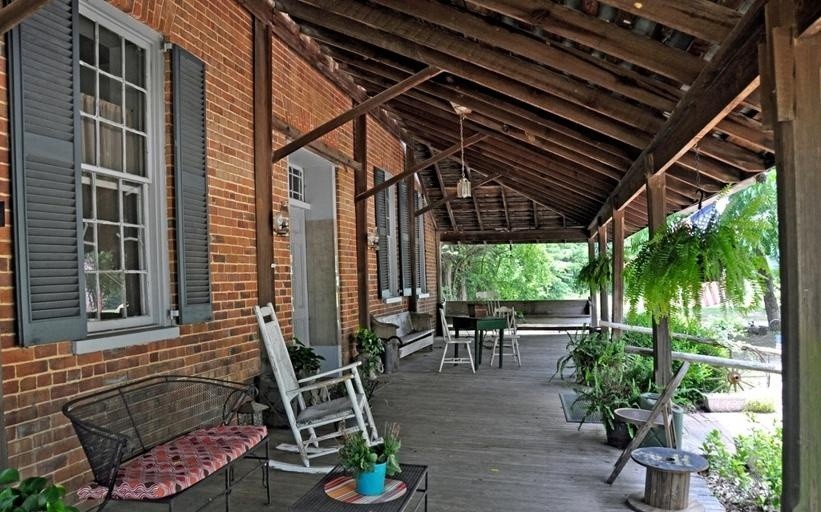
[558,389,606,424]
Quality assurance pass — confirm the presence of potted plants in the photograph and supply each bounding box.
[545,326,610,391]
[353,326,385,393]
[568,347,660,445]
[336,419,403,495]
[619,215,776,326]
[252,332,328,434]
[571,242,642,299]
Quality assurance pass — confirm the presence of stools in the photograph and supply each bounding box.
[626,444,709,512]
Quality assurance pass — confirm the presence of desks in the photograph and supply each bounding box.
[284,457,431,512]
[451,314,507,369]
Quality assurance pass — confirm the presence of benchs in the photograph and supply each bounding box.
[61,372,272,511]
[372,307,436,376]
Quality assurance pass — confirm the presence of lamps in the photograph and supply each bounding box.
[366,225,384,255]
[272,198,292,238]
[449,102,474,200]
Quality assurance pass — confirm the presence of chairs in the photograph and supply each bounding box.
[490,306,523,367]
[442,294,594,328]
[604,360,691,487]
[250,302,383,475]
[437,308,477,375]
